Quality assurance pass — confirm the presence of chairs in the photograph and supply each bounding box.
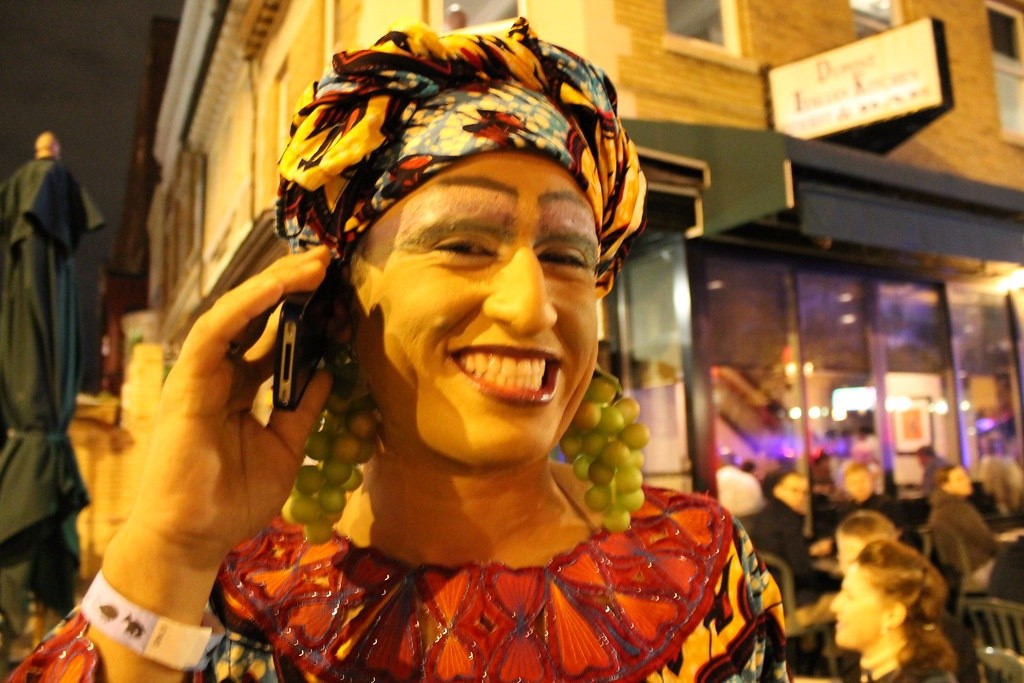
[968,598,1024,683]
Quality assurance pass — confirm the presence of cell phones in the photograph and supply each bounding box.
[273,266,328,410]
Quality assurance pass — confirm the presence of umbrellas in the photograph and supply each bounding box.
[0,130,89,653]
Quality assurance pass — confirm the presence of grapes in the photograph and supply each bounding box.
[282,374,382,543]
[557,363,651,530]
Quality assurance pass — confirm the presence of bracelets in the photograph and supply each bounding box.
[80,571,212,671]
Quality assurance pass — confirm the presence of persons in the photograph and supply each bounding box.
[747,445,1024,682]
[0,18,794,683]
